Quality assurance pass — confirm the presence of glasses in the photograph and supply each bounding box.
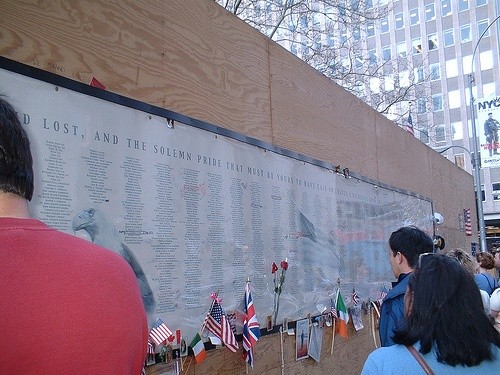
[419,252,436,269]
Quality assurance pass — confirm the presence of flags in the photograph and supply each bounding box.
[146,280,259,368]
[331,286,389,340]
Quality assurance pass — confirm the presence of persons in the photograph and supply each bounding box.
[0,98,148,375]
[362,227,500,375]
